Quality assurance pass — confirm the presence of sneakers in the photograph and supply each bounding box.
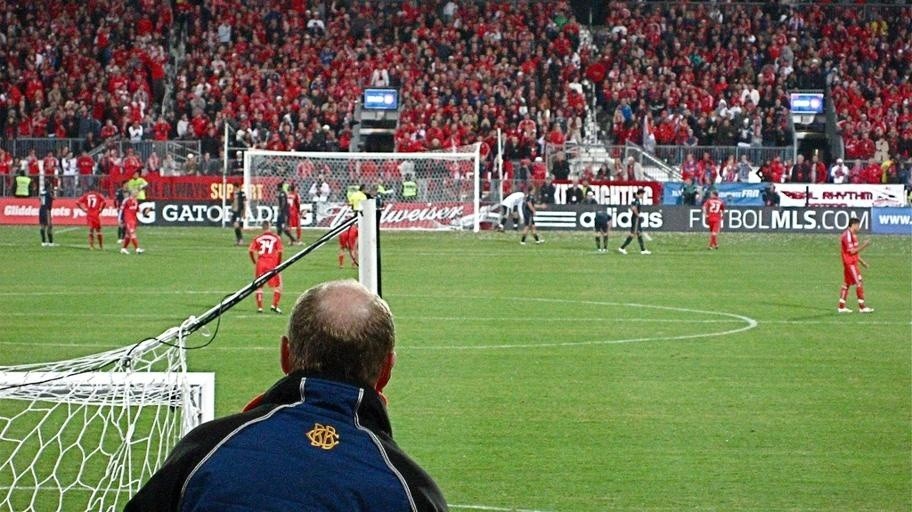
[40,242,61,248]
[520,241,527,246]
[535,240,545,245]
[617,247,628,256]
[270,304,284,315]
[597,248,608,252]
[256,307,264,313]
[837,306,854,313]
[117,238,123,244]
[640,248,652,256]
[858,306,875,314]
[120,247,146,256]
[287,240,307,248]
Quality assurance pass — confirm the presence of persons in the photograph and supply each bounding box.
[835,217,874,313]
[125,276,449,511]
[617,186,652,255]
[494,190,527,233]
[700,191,725,250]
[347,184,367,212]
[593,212,612,253]
[275,183,296,245]
[117,189,153,255]
[338,219,359,269]
[286,185,307,246]
[113,179,132,244]
[520,184,545,246]
[248,220,283,313]
[230,181,247,247]
[37,179,60,247]
[1,1,911,208]
[75,183,108,253]
